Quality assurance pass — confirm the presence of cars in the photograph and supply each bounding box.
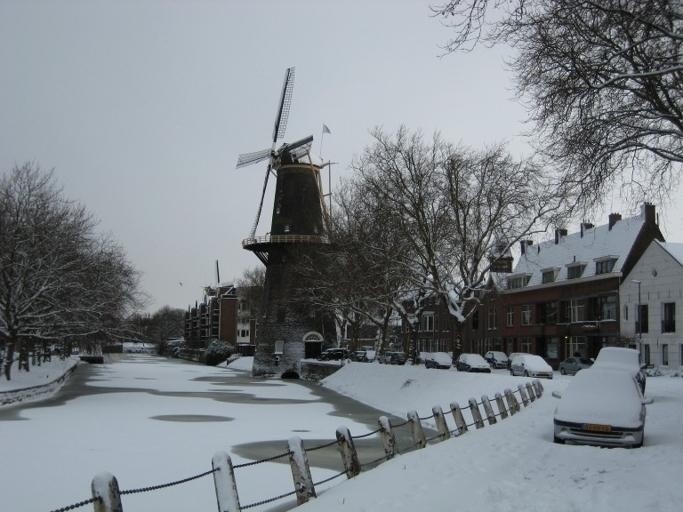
[485,351,509,369]
[551,369,654,448]
[589,346,650,396]
[425,353,451,369]
[559,356,594,376]
[456,353,491,372]
[510,354,553,380]
